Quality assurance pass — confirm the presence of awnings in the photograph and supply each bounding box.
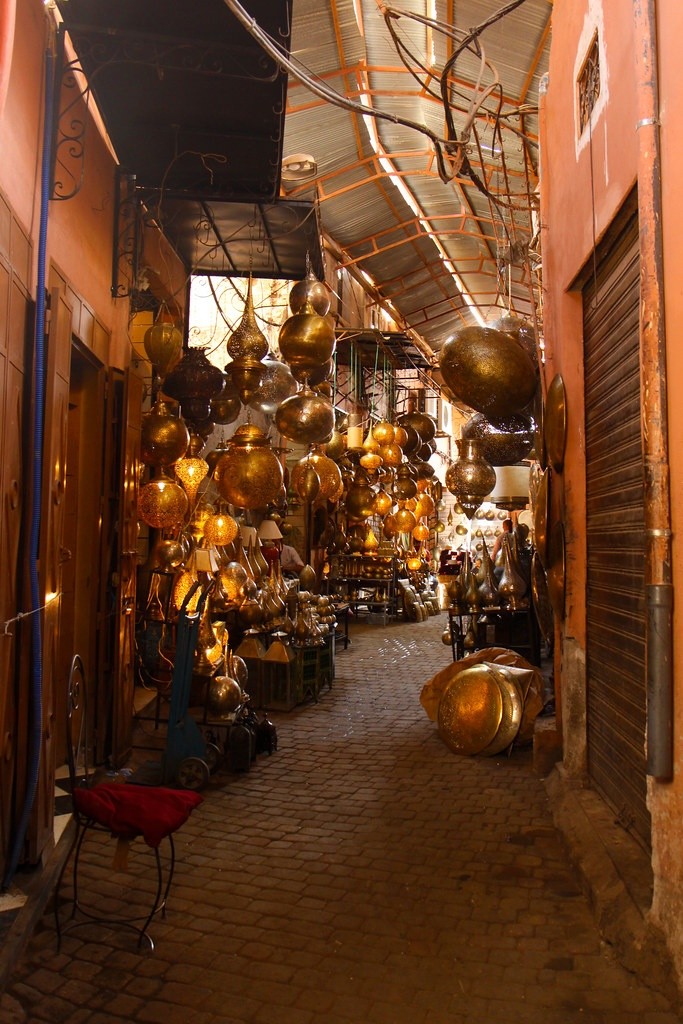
[56,0,293,202]
[137,187,327,280]
[333,328,430,371]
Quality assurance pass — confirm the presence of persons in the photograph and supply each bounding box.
[471,559,482,575]
[492,520,528,582]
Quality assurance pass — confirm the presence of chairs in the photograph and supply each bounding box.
[52,654,179,956]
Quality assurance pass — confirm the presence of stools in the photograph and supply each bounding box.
[329,602,350,650]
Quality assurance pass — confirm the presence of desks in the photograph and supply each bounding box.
[449,606,537,668]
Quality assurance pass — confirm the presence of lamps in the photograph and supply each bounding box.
[281,153,318,181]
[138,247,569,715]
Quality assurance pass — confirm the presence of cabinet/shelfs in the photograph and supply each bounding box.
[291,630,335,704]
[329,553,399,626]
